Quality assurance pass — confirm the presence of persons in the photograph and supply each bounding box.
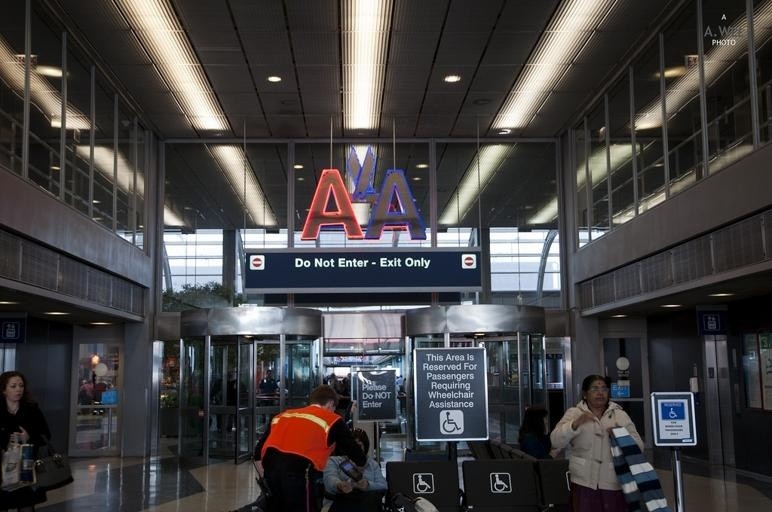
[1,371,76,511]
[320,426,389,511]
[519,405,553,458]
[211,369,250,435]
[550,375,645,511]
[259,369,278,426]
[321,370,409,429]
[255,386,369,511]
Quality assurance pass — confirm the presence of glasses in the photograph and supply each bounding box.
[588,387,609,393]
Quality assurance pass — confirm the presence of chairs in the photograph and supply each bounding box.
[308,439,570,512]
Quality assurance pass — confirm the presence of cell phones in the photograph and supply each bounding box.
[338,459,362,482]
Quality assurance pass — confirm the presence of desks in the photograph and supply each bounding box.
[77,405,117,453]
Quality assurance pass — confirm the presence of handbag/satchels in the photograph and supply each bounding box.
[413,496,438,512]
[35,433,74,490]
[0,432,37,492]
[391,491,415,512]
